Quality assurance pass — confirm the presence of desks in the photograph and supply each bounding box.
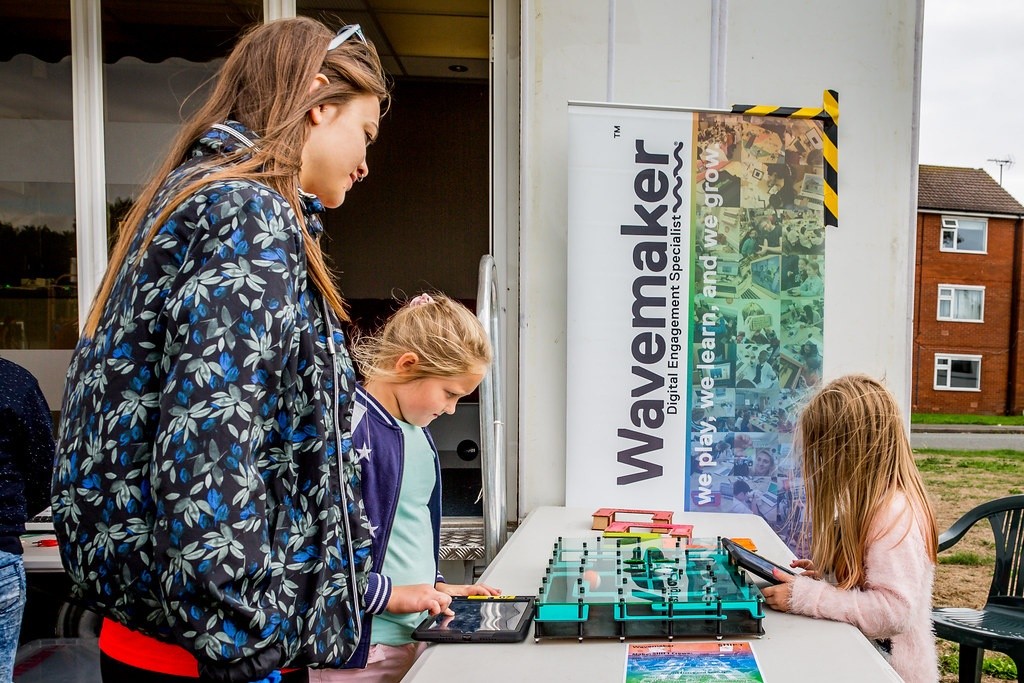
[400,505,911,683]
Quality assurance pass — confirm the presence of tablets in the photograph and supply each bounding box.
[412,595,535,642]
[722,538,800,584]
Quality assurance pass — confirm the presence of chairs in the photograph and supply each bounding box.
[928,495,1024,683]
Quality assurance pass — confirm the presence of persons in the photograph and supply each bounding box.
[757,374,940,683]
[696,109,823,529]
[56,15,390,682]
[1,359,55,681]
[305,288,502,682]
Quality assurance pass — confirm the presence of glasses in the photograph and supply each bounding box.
[327,24,367,51]
